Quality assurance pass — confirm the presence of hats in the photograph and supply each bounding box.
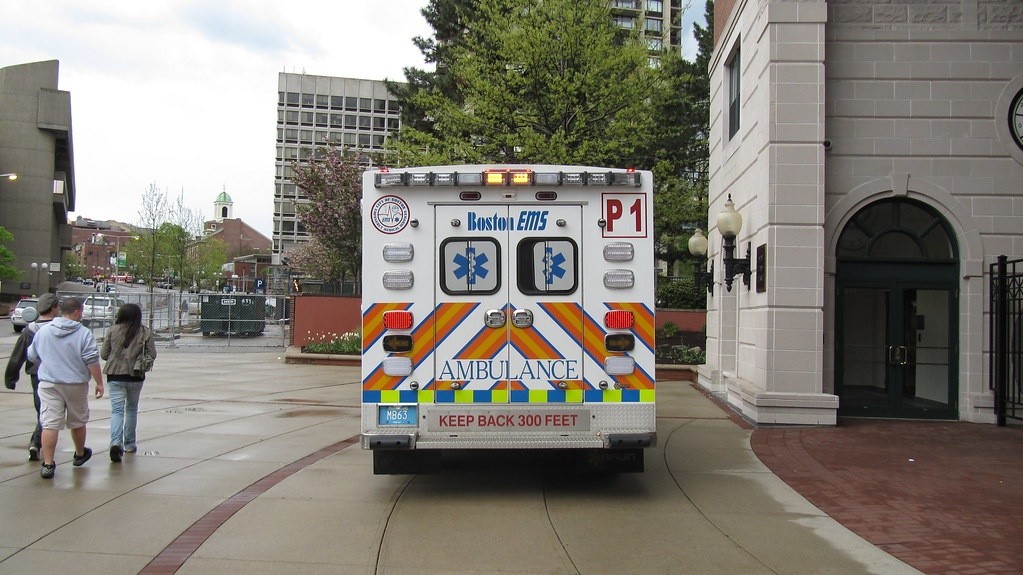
[38,293,59,314]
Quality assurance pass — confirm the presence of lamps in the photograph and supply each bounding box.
[717,193,752,292]
[688,215,714,297]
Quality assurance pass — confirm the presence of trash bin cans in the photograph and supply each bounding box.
[199,294,268,337]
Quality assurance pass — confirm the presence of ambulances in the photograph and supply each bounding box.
[359,164,657,474]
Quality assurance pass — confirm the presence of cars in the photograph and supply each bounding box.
[76,274,199,298]
[57,294,85,305]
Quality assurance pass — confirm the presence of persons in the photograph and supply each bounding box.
[4,292,59,460]
[181,299,188,319]
[106,285,111,292]
[100,302,157,462]
[28,297,104,478]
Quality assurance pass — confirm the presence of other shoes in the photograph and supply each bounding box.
[41,461,56,477]
[110,444,122,462]
[29,447,41,461]
[125,447,137,453]
[73,448,92,466]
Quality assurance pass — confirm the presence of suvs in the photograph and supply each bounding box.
[11,298,39,332]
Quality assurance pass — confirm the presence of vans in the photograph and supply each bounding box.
[81,296,127,328]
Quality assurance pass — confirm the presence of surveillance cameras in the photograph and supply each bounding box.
[823,140,833,149]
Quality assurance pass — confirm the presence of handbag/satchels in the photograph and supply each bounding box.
[133,327,153,374]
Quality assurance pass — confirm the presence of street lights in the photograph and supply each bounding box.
[31,262,48,297]
[96,233,141,289]
